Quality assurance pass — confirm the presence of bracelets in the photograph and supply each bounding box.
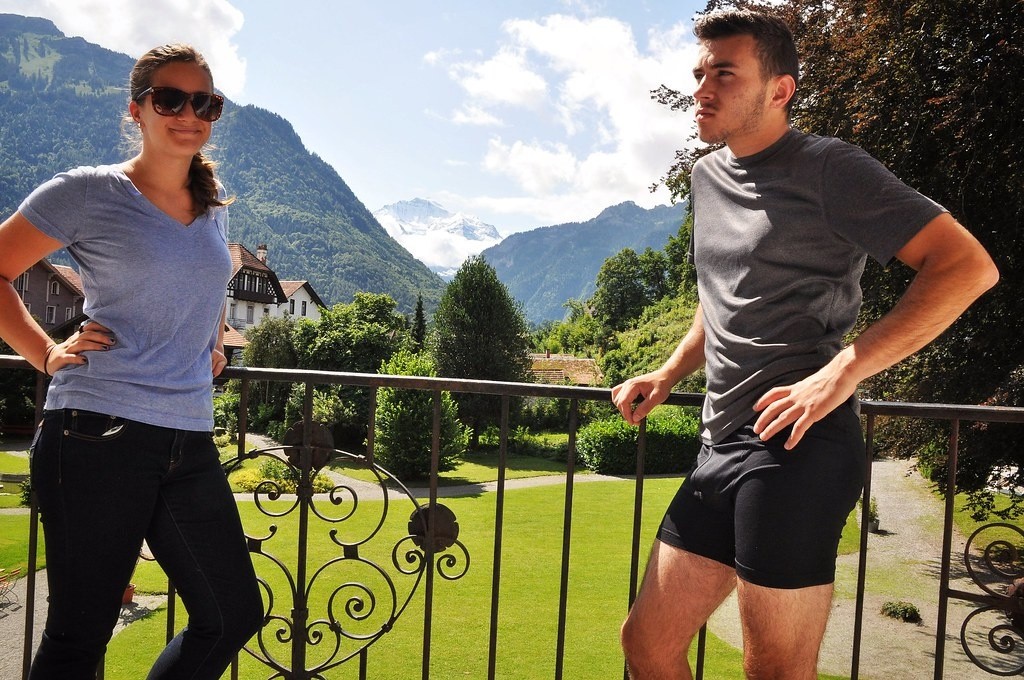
[44,341,57,378]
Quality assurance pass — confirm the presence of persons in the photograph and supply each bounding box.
[610,6,1003,679]
[0,45,269,679]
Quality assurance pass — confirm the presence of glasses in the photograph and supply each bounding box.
[136,86,224,122]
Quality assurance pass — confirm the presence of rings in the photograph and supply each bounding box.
[78,325,85,333]
